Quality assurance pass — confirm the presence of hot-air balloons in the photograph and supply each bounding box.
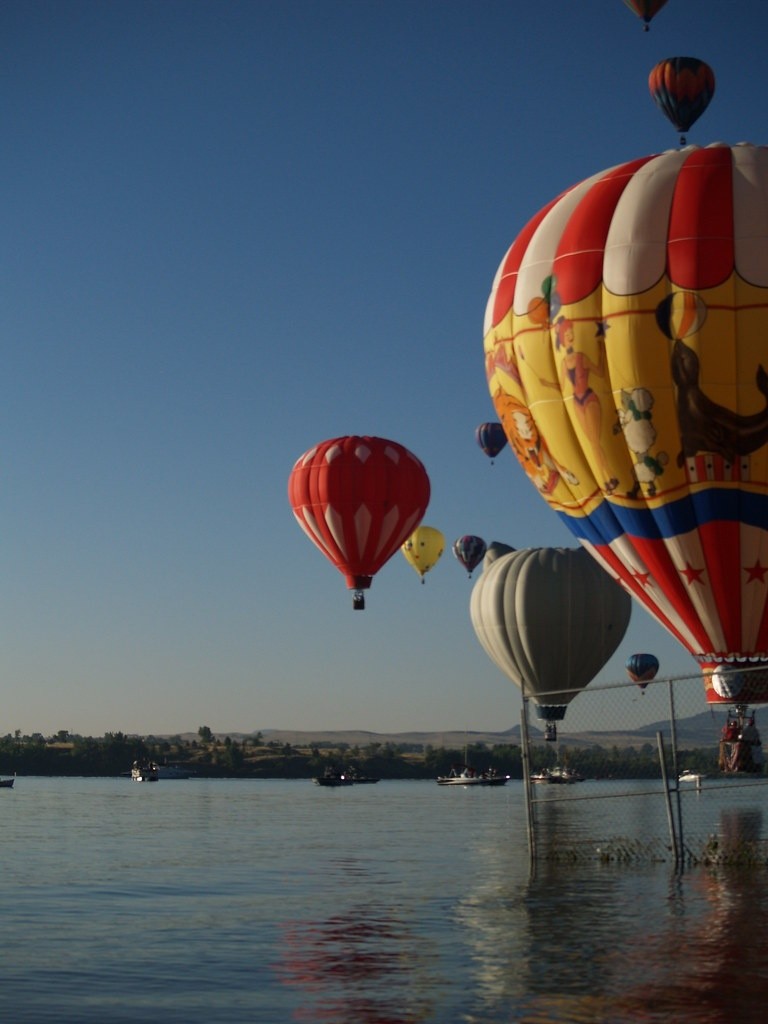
[401,526,444,584]
[474,423,507,465]
[649,57,716,145]
[452,535,487,579]
[625,654,659,695]
[624,0,667,32]
[289,436,431,610]
[483,140,768,775]
[470,545,632,742]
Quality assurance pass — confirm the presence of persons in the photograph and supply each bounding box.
[149,761,153,770]
[742,719,760,742]
[546,725,556,732]
[722,719,740,742]
[469,768,476,778]
[355,595,363,601]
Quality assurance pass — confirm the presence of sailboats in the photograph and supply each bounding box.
[437,743,511,785]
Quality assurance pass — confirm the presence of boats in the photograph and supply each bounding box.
[132,761,160,782]
[352,776,381,784]
[679,770,708,782]
[530,768,584,784]
[312,765,354,786]
[0,778,14,787]
